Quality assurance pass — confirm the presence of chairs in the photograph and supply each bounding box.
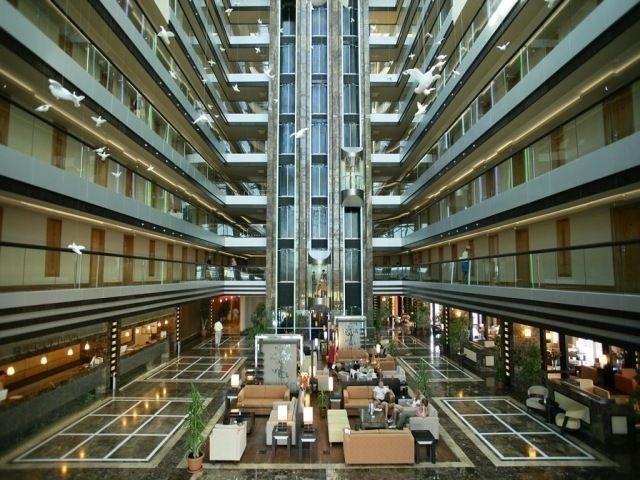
[209,421,247,465]
[525,385,585,436]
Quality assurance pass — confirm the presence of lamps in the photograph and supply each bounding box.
[303,407,314,435]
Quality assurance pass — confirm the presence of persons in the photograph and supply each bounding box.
[226,306,231,323]
[550,349,557,370]
[321,269,327,285]
[460,245,471,284]
[233,306,239,324]
[214,317,224,346]
[133,94,143,119]
[0,369,7,392]
[207,255,212,264]
[628,373,640,441]
[311,271,316,292]
[231,257,238,266]
[326,339,429,430]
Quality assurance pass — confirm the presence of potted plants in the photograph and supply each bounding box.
[313,386,332,418]
[178,381,212,474]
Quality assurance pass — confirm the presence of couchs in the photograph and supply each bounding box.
[236,384,298,449]
[334,348,439,464]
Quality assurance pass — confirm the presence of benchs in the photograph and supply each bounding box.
[326,409,351,447]
